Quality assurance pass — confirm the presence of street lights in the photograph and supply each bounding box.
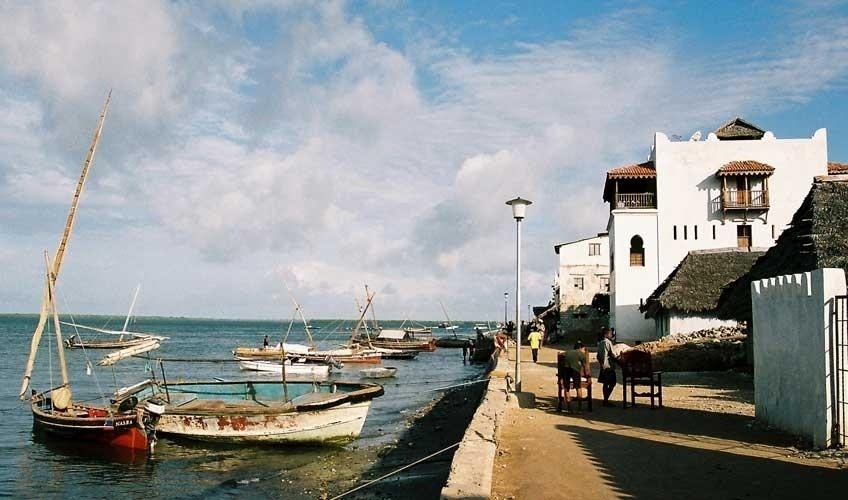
[505,195,533,393]
[528,303,533,325]
[503,289,512,351]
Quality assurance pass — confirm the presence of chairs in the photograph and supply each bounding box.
[556,348,663,415]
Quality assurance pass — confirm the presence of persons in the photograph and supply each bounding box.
[263,335,269,348]
[560,339,587,416]
[507,321,515,338]
[596,327,619,407]
[528,319,536,332]
[475,326,484,341]
[537,318,546,349]
[495,331,507,350]
[507,336,517,346]
[527,326,542,363]
[520,319,526,344]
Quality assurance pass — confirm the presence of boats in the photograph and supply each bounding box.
[106,374,385,449]
[303,322,493,360]
[31,387,166,462]
[235,356,344,377]
[62,334,171,349]
[360,366,398,379]
[231,342,382,364]
